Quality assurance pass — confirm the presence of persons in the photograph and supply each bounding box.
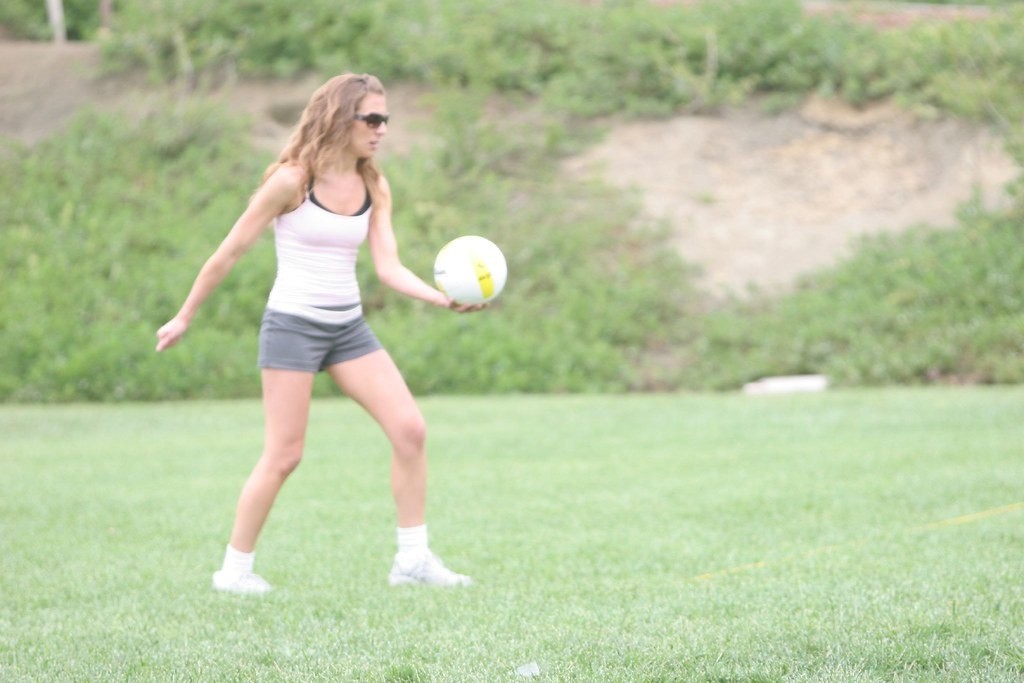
[157,74,491,595]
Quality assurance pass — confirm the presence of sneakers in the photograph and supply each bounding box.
[213,571,270,597]
[390,553,471,590]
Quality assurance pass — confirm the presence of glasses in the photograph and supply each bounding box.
[354,113,390,129]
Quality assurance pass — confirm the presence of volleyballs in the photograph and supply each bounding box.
[435,234,507,306]
